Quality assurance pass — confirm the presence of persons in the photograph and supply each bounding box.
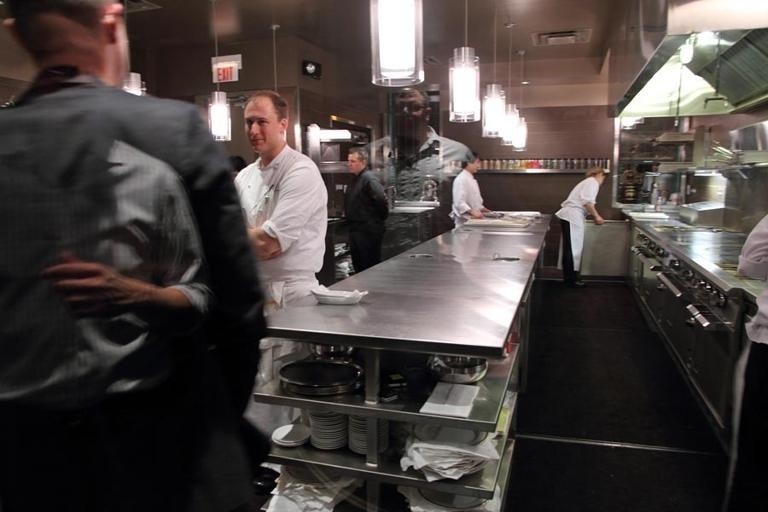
[5,141,210,509]
[0,0,280,511]
[345,148,389,271]
[363,86,473,198]
[449,150,491,228]
[723,208,768,512]
[555,167,606,288]
[233,95,328,436]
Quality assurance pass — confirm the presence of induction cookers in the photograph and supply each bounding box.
[628,225,763,333]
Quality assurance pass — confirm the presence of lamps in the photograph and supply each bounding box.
[370,1,424,87]
[206,0,234,143]
[450,0,480,124]
[482,7,529,151]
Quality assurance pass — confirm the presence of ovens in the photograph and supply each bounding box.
[620,245,742,436]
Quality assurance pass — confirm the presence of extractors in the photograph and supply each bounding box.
[606,1,768,119]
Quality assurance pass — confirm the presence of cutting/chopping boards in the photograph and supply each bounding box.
[462,218,531,227]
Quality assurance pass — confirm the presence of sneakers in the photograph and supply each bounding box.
[567,282,591,296]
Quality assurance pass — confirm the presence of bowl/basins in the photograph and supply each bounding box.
[428,357,487,384]
[298,342,357,357]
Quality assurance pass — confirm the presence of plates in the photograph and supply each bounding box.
[270,425,310,447]
[312,287,366,305]
[417,488,488,509]
[282,465,341,484]
[412,424,488,446]
[309,411,389,454]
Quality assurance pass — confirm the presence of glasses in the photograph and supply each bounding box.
[397,103,424,111]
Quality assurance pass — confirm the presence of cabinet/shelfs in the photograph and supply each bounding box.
[253,210,554,512]
